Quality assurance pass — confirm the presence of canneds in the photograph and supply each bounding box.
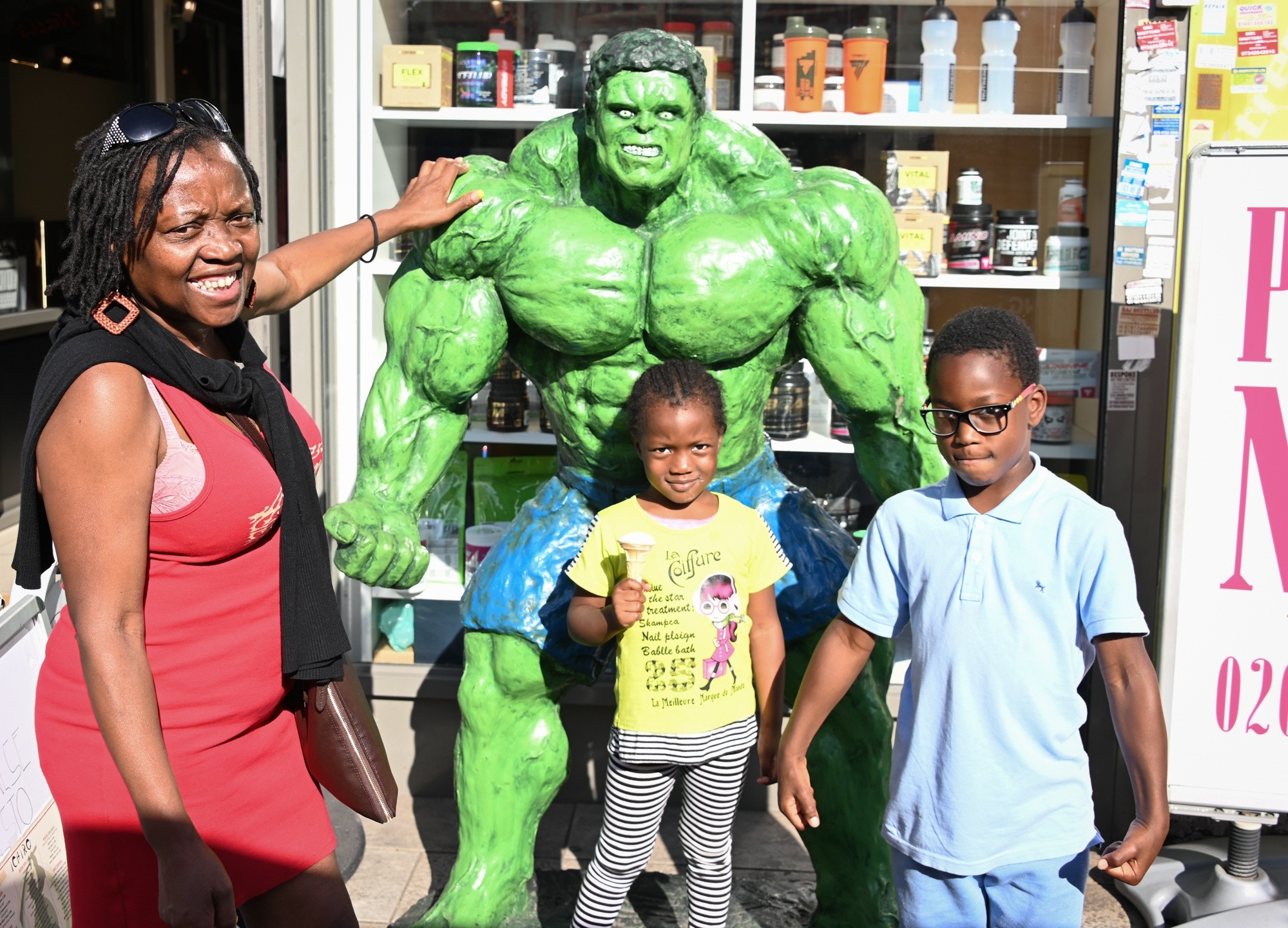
[763,361,811,440]
[454,40,500,108]
[946,203,1093,277]
[512,49,557,108]
[660,20,738,110]
[465,525,501,586]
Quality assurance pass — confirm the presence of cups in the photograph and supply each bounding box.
[841,17,889,113]
[784,16,828,112]
[456,30,576,108]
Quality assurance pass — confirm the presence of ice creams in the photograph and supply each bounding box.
[618,532,655,583]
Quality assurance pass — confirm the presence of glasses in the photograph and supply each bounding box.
[919,384,1037,437]
[98,98,232,161]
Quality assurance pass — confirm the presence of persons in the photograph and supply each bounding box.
[562,361,793,928]
[776,309,1170,927]
[33,102,486,928]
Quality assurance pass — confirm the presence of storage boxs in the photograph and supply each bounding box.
[335,2,1121,704]
[377,43,455,112]
[878,149,952,278]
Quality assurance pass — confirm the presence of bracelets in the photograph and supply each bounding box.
[358,214,378,264]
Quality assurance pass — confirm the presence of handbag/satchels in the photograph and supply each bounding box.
[294,648,398,827]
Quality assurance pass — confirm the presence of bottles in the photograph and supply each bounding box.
[831,403,853,443]
[920,1,959,113]
[1056,0,1097,115]
[978,0,1019,114]
[1042,178,1091,274]
[764,360,809,441]
[1031,391,1077,445]
[923,328,935,368]
[486,353,529,433]
[582,34,608,105]
[947,167,1038,274]
